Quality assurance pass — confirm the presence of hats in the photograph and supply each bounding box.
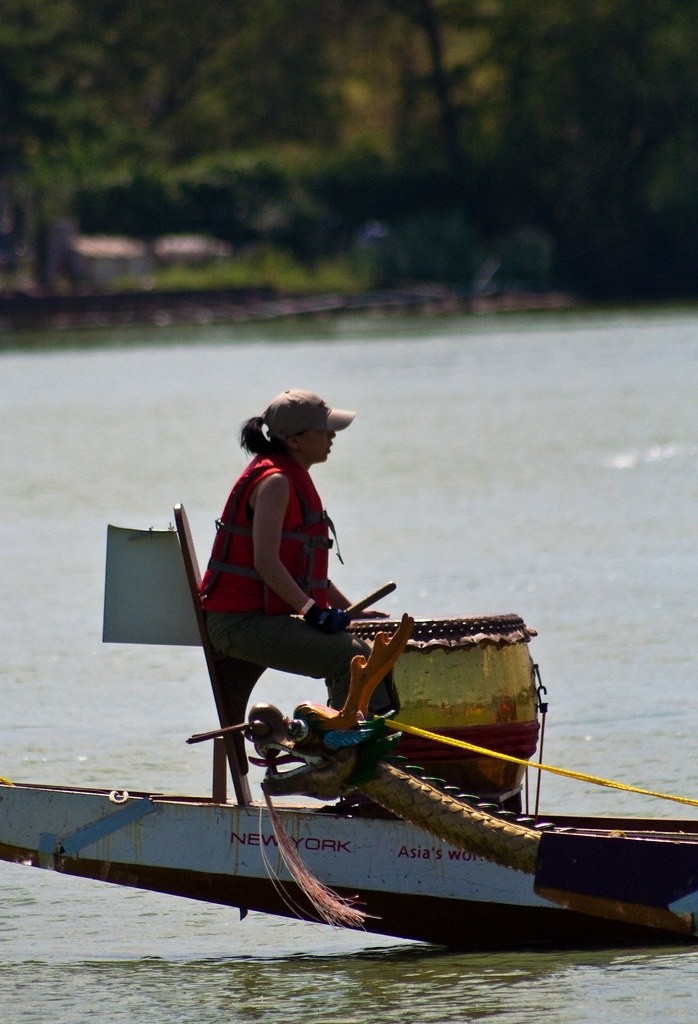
[264,390,357,437]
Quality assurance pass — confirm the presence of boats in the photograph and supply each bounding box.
[0,775,698,953]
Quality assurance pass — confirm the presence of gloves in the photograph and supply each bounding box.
[304,603,351,634]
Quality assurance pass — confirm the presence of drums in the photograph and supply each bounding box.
[334,612,541,795]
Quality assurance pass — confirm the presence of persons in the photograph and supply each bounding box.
[200,389,391,712]
